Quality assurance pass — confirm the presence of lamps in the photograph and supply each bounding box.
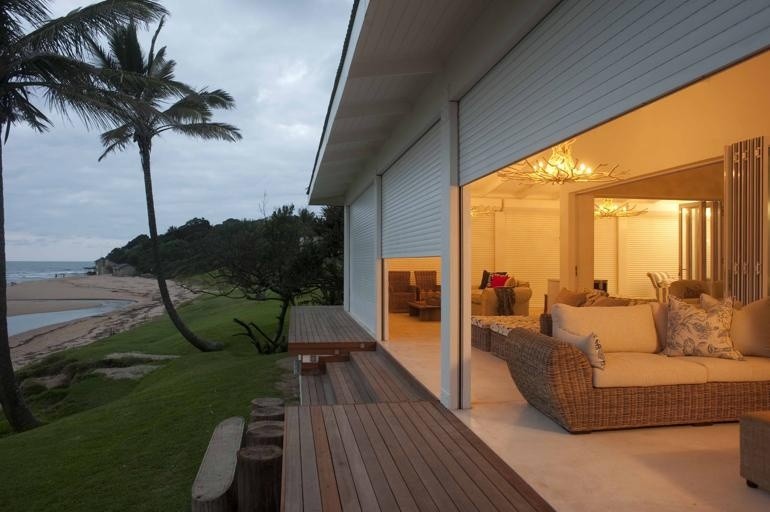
[496,137,650,222]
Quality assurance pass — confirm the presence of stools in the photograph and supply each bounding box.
[738,408,770,492]
[471,316,541,361]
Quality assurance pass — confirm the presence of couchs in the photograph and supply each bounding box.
[505,288,769,437]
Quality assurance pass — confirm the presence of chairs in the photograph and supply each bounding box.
[382,266,532,316]
[645,270,679,303]
